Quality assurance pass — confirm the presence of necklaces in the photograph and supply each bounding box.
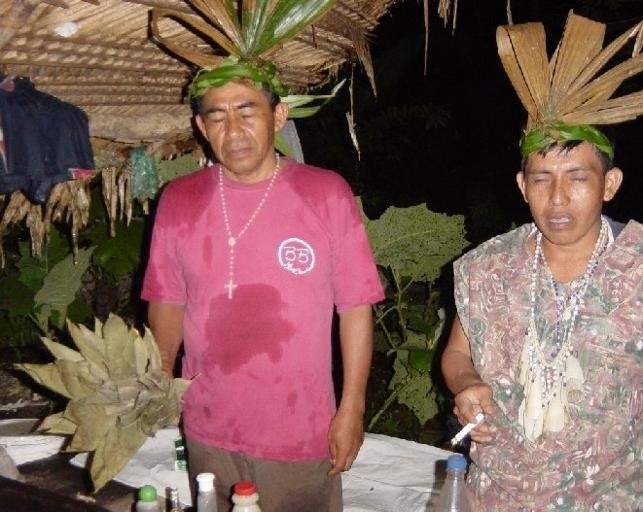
[513,212,610,440]
[216,153,280,299]
[532,217,609,359]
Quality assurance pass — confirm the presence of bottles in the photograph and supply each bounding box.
[436,455,473,512]
[136,485,161,512]
[231,482,262,512]
[196,472,218,512]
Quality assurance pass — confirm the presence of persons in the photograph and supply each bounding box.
[137,61,386,511]
[440,122,642,512]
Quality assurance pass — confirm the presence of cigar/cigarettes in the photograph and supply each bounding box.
[450,412,486,446]
